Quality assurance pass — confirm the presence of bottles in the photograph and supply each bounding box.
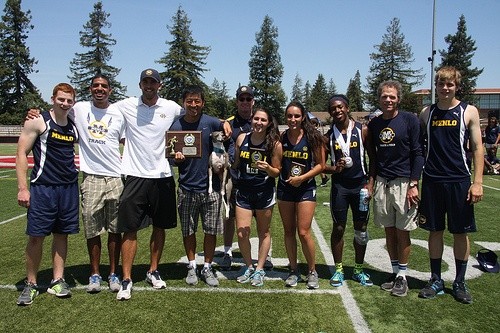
[359,188,368,211]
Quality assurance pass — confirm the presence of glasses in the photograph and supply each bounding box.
[238,97,254,102]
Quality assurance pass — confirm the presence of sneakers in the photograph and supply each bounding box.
[352,270,373,286]
[418,278,444,298]
[116,278,133,300]
[200,268,219,286]
[452,279,472,304]
[390,276,408,297]
[330,271,345,287]
[237,267,255,283]
[107,274,121,292]
[381,273,396,291]
[304,270,319,288]
[264,256,273,268]
[144,269,166,289]
[186,265,198,286]
[47,277,72,297]
[16,280,39,305]
[240,269,266,287]
[219,254,232,271]
[87,273,102,292]
[284,273,303,287]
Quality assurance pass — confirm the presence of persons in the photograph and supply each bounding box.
[234,107,283,286]
[310,118,329,187]
[25,74,127,294]
[485,117,500,156]
[221,87,275,271]
[279,102,325,289]
[418,66,484,303]
[16,84,81,305]
[483,148,500,175]
[364,80,425,297]
[110,69,233,301]
[322,95,376,287]
[166,86,235,287]
[165,135,178,152]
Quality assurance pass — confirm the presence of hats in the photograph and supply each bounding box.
[476,248,499,273]
[236,86,254,98]
[140,69,161,82]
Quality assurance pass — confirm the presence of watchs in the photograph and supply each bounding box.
[409,182,417,188]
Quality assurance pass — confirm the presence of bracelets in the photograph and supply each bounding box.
[223,120,228,123]
